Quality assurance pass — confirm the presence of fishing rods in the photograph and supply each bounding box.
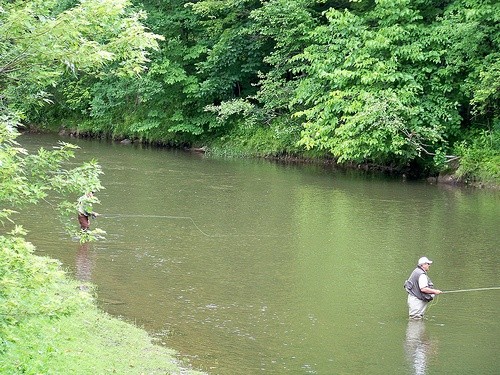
[92,214,193,219]
[440,287,500,294]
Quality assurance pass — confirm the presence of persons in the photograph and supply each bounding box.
[76,189,98,233]
[403,256,442,320]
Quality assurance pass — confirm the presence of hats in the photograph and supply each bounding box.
[417,256,433,265]
